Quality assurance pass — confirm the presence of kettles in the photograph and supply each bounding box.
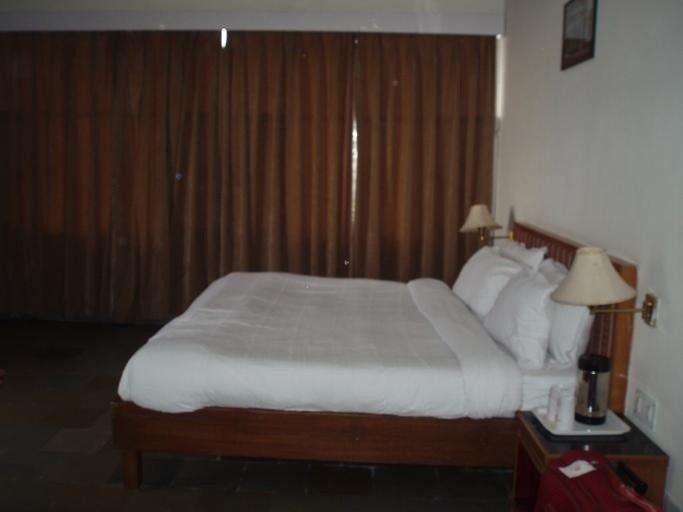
[573,353,612,426]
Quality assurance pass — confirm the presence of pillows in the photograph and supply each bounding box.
[452,241,595,370]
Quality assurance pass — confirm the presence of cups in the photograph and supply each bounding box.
[545,383,563,425]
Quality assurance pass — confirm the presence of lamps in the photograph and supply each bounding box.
[459,205,509,242]
[551,247,657,327]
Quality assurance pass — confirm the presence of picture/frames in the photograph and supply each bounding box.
[562,0,596,70]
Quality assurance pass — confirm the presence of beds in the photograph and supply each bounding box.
[110,222,637,490]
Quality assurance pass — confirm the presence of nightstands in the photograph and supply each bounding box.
[510,411,669,512]
